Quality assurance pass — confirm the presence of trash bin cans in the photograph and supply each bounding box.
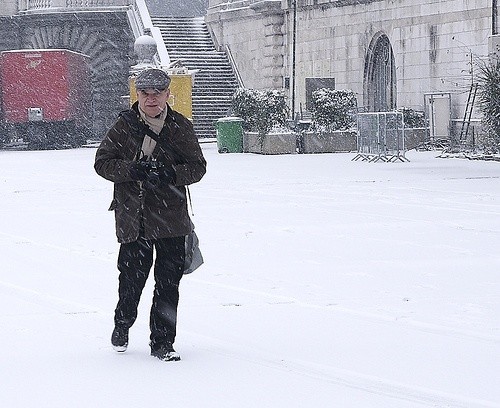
[216,117,245,153]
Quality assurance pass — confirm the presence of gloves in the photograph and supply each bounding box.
[130,161,174,191]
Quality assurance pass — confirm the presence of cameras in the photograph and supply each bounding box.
[139,161,164,189]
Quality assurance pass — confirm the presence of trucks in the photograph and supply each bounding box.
[2,47,95,153]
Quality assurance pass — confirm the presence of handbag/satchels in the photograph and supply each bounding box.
[184,218,203,274]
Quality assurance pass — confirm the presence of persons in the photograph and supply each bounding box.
[95,69,205,361]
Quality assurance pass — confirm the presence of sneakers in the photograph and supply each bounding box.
[110,325,129,351]
[150,340,180,362]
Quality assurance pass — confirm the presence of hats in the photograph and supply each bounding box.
[135,68,171,89]
